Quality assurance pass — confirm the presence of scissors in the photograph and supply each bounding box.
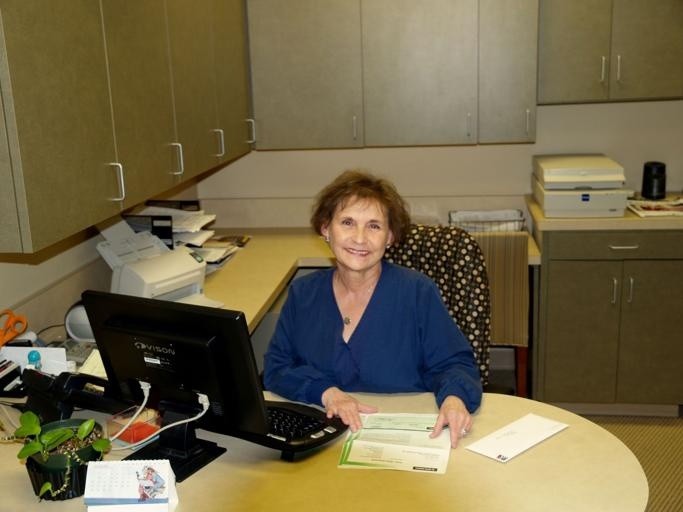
[0,309,28,349]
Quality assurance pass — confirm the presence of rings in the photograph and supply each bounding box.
[460,428,468,438]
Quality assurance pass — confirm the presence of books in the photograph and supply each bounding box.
[0,349,107,436]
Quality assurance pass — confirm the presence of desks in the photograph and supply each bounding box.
[1,228,651,512]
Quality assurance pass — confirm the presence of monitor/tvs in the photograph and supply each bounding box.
[80,287,270,485]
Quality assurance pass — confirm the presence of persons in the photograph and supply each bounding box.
[263,169,482,449]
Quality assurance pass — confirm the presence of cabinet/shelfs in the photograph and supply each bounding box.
[533,232,683,417]
[537,1,683,105]
[248,1,537,152]
[0,0,253,266]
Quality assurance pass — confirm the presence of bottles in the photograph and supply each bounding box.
[640,161,666,200]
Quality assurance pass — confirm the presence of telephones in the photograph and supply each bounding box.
[22,369,113,427]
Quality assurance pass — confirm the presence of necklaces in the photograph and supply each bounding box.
[334,270,378,326]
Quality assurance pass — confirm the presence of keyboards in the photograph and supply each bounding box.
[265,400,348,463]
